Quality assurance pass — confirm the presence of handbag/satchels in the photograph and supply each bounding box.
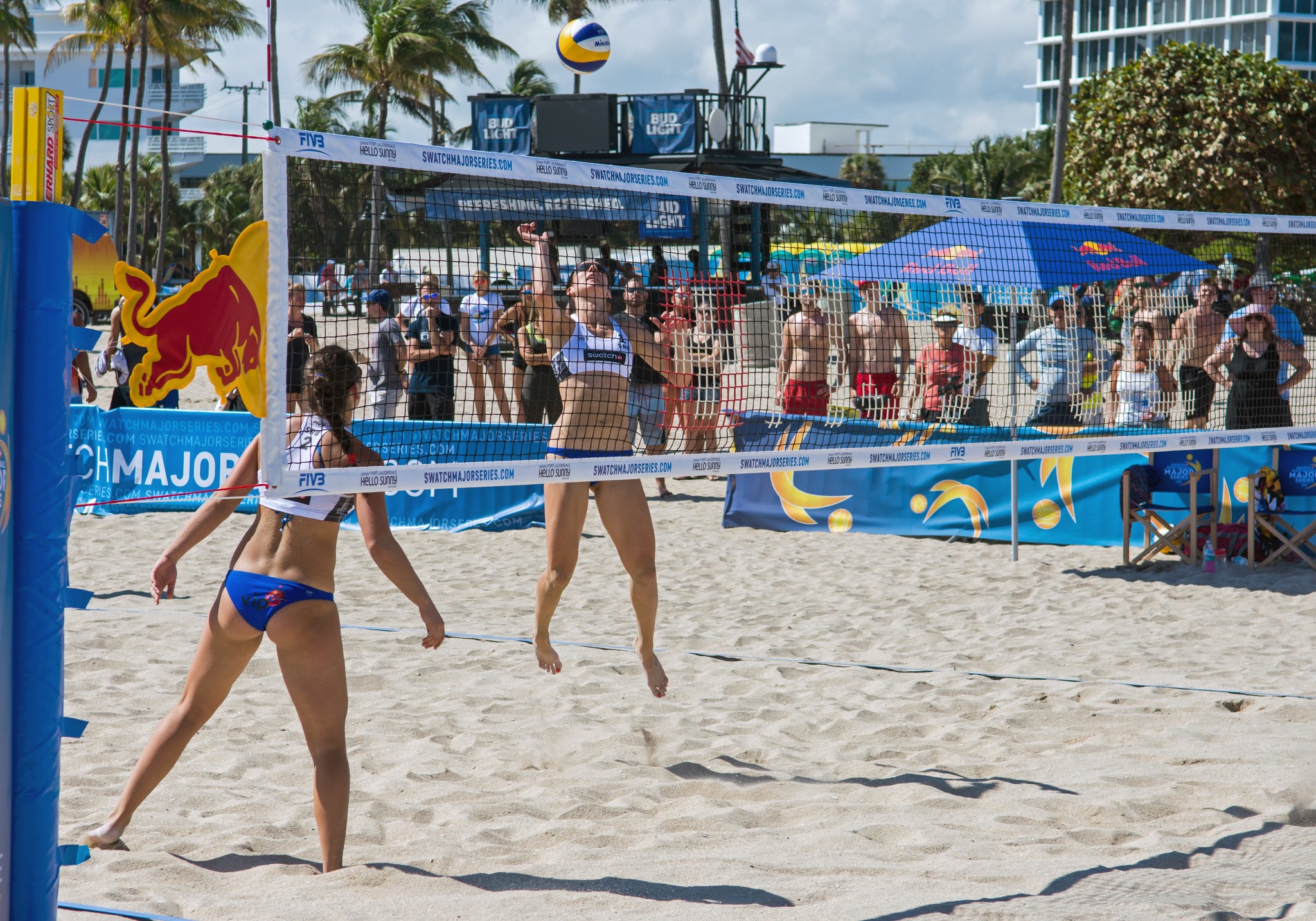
[1183,522,1263,562]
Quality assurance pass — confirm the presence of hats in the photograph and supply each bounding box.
[962,292,985,306]
[359,289,390,306]
[327,260,335,265]
[931,316,960,324]
[1228,303,1279,336]
[858,279,878,288]
[1049,291,1073,306]
[354,259,365,266]
[384,261,393,267]
[1244,270,1280,303]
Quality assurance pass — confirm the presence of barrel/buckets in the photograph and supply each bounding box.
[817,291,851,344]
[731,302,782,367]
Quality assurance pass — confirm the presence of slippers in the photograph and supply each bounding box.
[660,492,677,499]
[707,475,721,481]
[673,475,692,480]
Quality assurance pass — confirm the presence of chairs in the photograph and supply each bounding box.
[1123,448,1219,565]
[1248,444,1316,569]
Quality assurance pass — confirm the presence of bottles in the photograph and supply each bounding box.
[1142,390,1150,411]
[1214,548,1227,571]
[1231,555,1257,567]
[899,409,921,423]
[1202,540,1216,573]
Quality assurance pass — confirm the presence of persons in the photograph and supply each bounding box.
[70,292,249,412]
[597,243,1316,492]
[84,345,443,871]
[284,231,571,426]
[518,222,667,700]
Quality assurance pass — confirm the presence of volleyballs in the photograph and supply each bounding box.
[555,18,610,74]
[1154,524,1186,555]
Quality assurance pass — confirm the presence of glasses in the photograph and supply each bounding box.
[1246,314,1263,322]
[767,268,777,273]
[964,298,981,305]
[1050,303,1071,311]
[520,290,532,296]
[473,280,487,285]
[1262,285,1278,292]
[573,262,715,316]
[1133,282,1150,289]
[802,288,814,296]
[935,321,955,327]
[1075,311,1082,317]
[420,293,440,300]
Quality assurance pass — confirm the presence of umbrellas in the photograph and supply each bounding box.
[818,198,1224,561]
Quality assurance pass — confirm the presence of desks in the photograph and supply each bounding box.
[372,283,419,317]
